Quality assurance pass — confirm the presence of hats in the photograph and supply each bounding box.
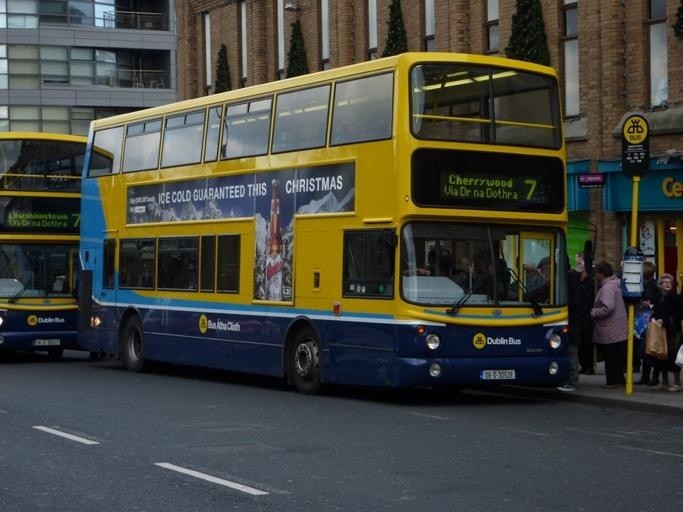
[658,273,673,283]
[535,255,551,270]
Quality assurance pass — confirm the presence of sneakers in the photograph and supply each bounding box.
[556,384,575,392]
[632,378,680,392]
[601,381,618,389]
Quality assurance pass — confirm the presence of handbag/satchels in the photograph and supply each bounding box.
[644,322,669,359]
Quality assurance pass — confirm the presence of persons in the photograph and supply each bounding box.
[403,247,545,301]
[527,249,683,393]
